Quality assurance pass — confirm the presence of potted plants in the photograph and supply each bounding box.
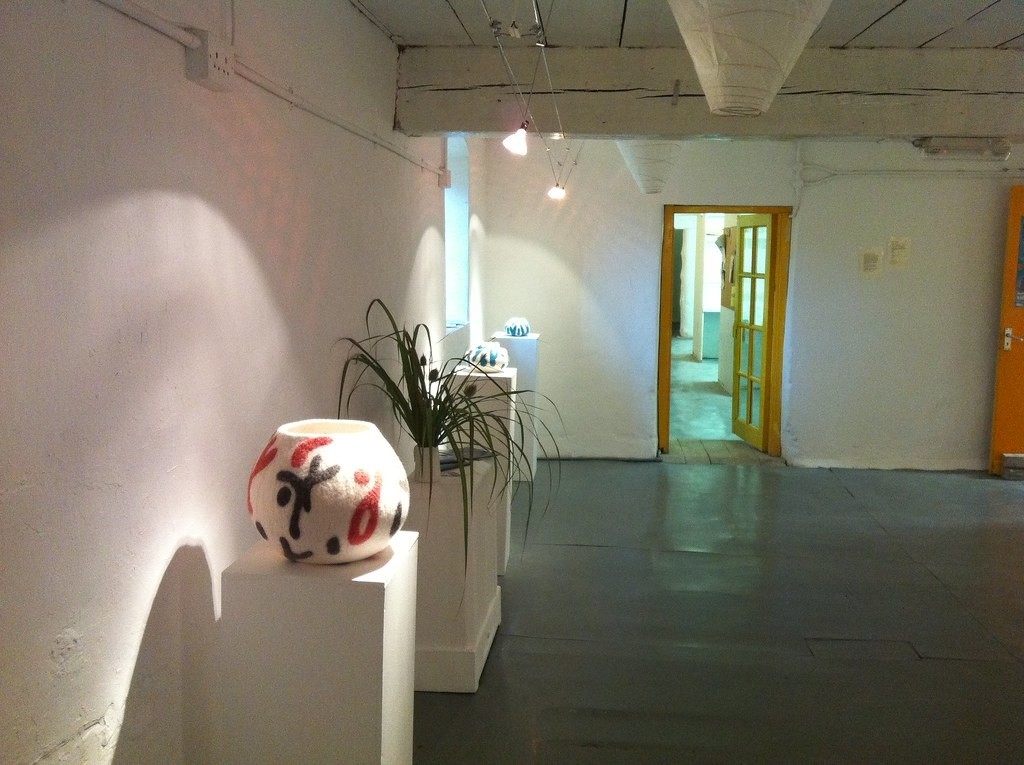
[335,294,560,520]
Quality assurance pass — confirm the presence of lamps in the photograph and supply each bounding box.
[499,41,547,156]
[545,140,585,200]
